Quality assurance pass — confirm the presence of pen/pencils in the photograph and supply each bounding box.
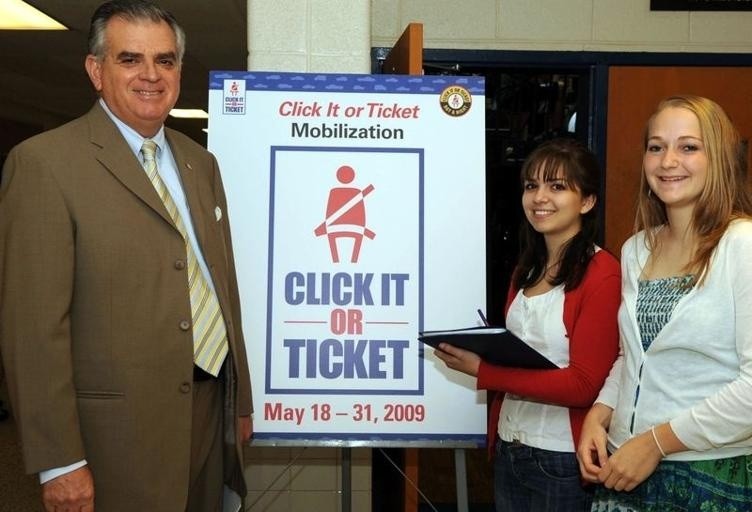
[477,309,490,327]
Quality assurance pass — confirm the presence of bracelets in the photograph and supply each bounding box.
[646,424,670,462]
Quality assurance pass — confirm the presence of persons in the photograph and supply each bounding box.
[2,0,254,512]
[432,131,622,511]
[572,94,750,510]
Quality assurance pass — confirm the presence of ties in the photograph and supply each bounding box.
[137,140,230,379]
